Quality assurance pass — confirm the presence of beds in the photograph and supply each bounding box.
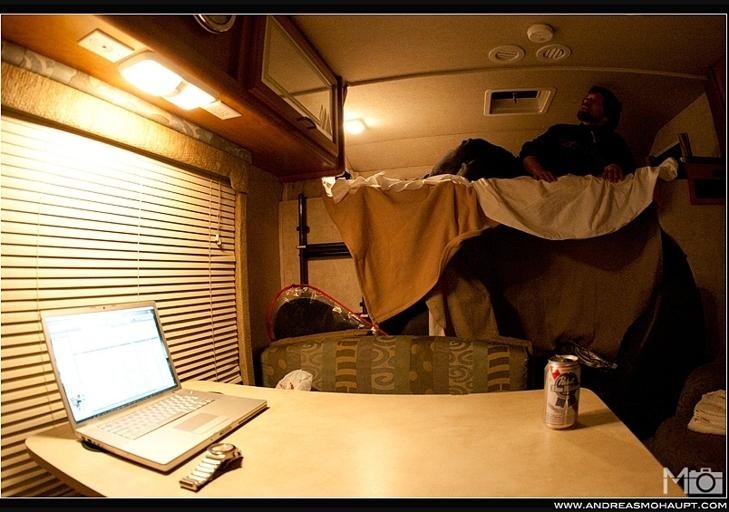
[318,130,725,205]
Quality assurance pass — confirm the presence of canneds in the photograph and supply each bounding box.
[543,354,579,430]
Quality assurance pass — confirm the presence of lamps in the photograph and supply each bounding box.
[117,50,221,111]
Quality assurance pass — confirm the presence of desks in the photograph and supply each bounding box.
[22,374,691,497]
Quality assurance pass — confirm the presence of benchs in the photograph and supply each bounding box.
[259,331,533,394]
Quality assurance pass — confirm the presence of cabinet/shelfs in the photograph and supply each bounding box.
[236,15,345,173]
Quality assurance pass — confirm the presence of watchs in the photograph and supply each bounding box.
[181,442,245,491]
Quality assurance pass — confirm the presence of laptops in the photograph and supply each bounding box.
[38,300,266,471]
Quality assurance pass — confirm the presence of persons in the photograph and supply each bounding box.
[423,88,628,181]
